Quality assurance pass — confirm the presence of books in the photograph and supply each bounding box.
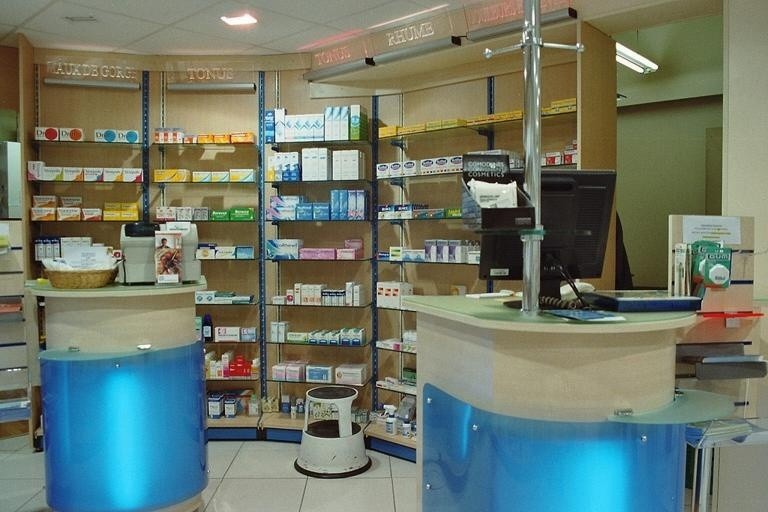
[684,418,752,445]
[681,353,762,363]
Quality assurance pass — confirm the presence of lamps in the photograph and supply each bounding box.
[614,40,658,76]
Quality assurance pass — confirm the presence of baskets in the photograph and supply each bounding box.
[44,267,118,289]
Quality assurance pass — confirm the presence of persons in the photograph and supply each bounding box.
[156,238,169,248]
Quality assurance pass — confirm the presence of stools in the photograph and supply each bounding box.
[295,386,371,480]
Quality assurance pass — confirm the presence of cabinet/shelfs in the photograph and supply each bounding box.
[1,21,621,463]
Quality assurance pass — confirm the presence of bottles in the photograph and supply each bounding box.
[202,315,214,342]
[402,419,412,439]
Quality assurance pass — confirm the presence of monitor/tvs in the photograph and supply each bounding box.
[480,169,618,310]
